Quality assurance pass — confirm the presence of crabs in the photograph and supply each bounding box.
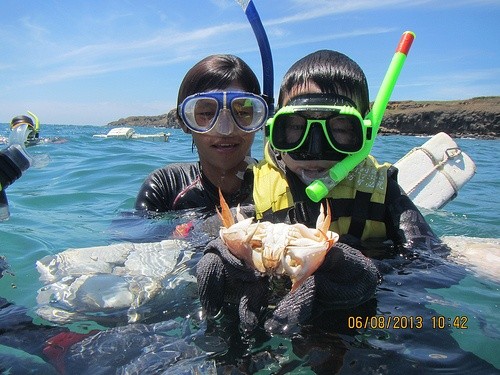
[213,186,337,293]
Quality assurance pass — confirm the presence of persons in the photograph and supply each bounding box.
[241,49,445,261]
[137,54,266,217]
[11,115,40,141]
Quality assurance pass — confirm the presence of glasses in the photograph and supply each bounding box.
[177,90,269,134]
[264,92,371,163]
